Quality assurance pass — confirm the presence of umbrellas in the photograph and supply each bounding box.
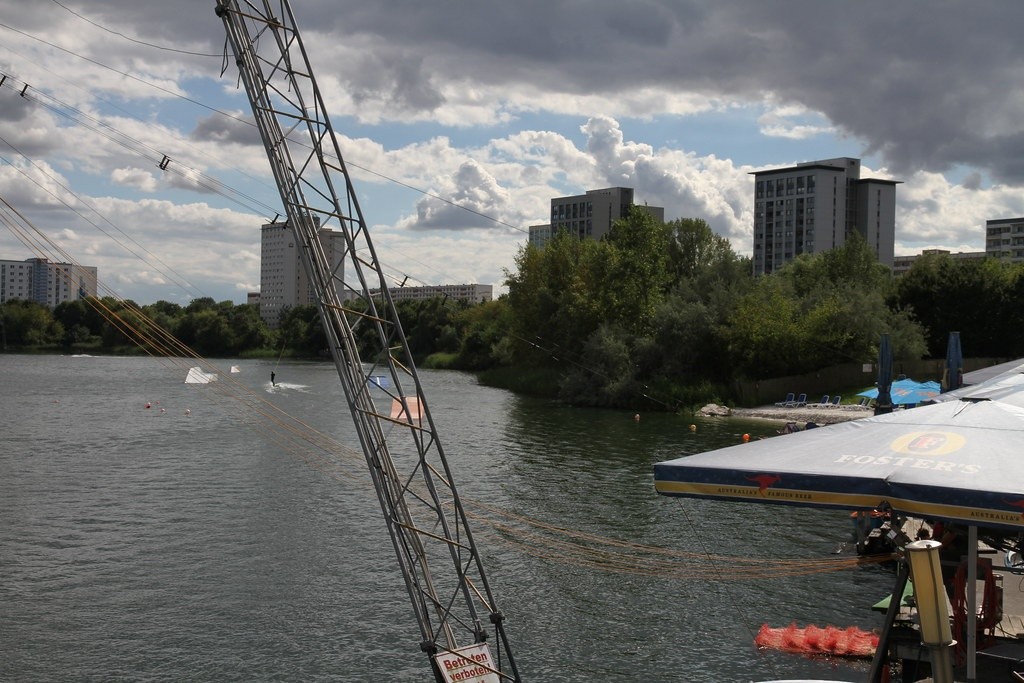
[873,334,893,415]
[939,333,962,394]
[655,358,1024,683]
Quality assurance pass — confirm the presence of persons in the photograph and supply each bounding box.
[271,372,275,386]
[940,530,967,614]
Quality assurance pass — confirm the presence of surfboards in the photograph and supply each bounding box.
[274,385,279,387]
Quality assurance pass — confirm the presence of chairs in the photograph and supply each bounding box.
[892,404,899,412]
[775,393,873,410]
